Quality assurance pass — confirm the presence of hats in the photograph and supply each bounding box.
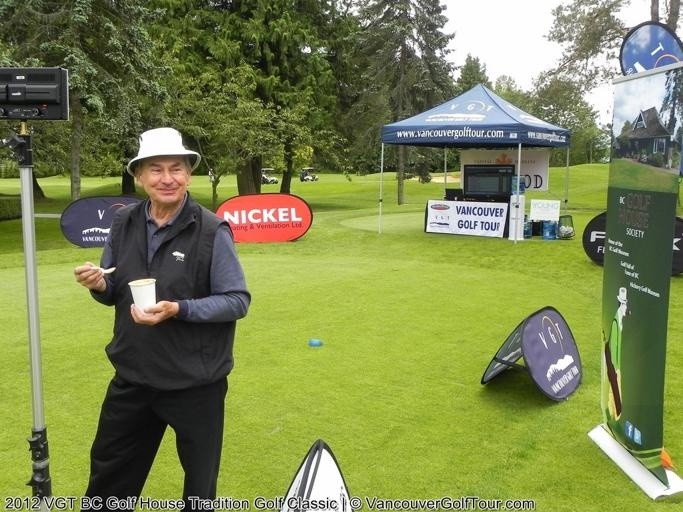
[616,287,628,304]
[127,127,201,176]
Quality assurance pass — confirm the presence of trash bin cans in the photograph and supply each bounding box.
[559,216,576,239]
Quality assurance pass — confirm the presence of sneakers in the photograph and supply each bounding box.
[605,408,624,433]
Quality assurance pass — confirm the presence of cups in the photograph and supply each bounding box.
[127,278,157,316]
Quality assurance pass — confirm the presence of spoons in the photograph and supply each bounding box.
[90,266,116,274]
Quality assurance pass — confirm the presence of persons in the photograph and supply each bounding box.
[71,126,252,512]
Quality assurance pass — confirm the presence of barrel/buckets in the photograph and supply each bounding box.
[512,175,525,195]
[542,222,557,240]
[523,216,533,238]
